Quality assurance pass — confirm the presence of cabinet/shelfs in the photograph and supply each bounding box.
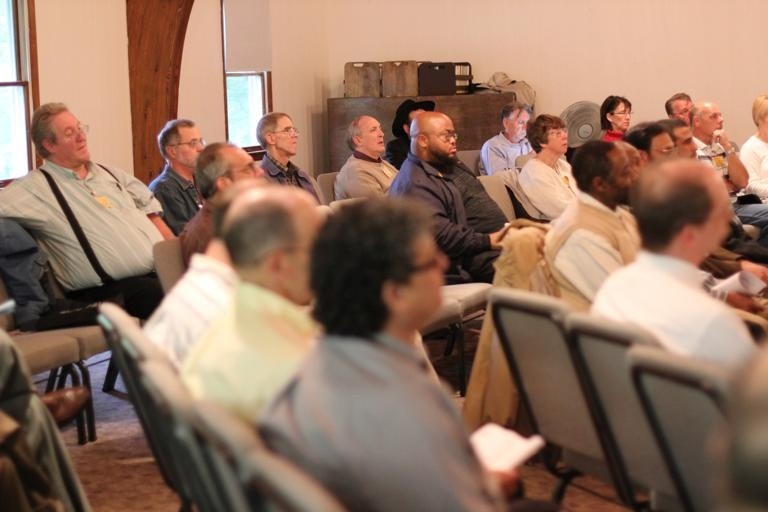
[380,60,432,98]
[344,60,384,98]
[418,60,473,97]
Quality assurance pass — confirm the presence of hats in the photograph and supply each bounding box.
[392,100,436,136]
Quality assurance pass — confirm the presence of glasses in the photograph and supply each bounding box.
[175,138,206,148]
[430,134,457,142]
[269,128,299,138]
[46,125,90,138]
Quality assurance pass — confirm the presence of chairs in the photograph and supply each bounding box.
[0,149,767,510]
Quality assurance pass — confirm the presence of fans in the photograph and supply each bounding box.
[556,101,604,148]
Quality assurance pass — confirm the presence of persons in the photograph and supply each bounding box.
[0,102,175,320]
